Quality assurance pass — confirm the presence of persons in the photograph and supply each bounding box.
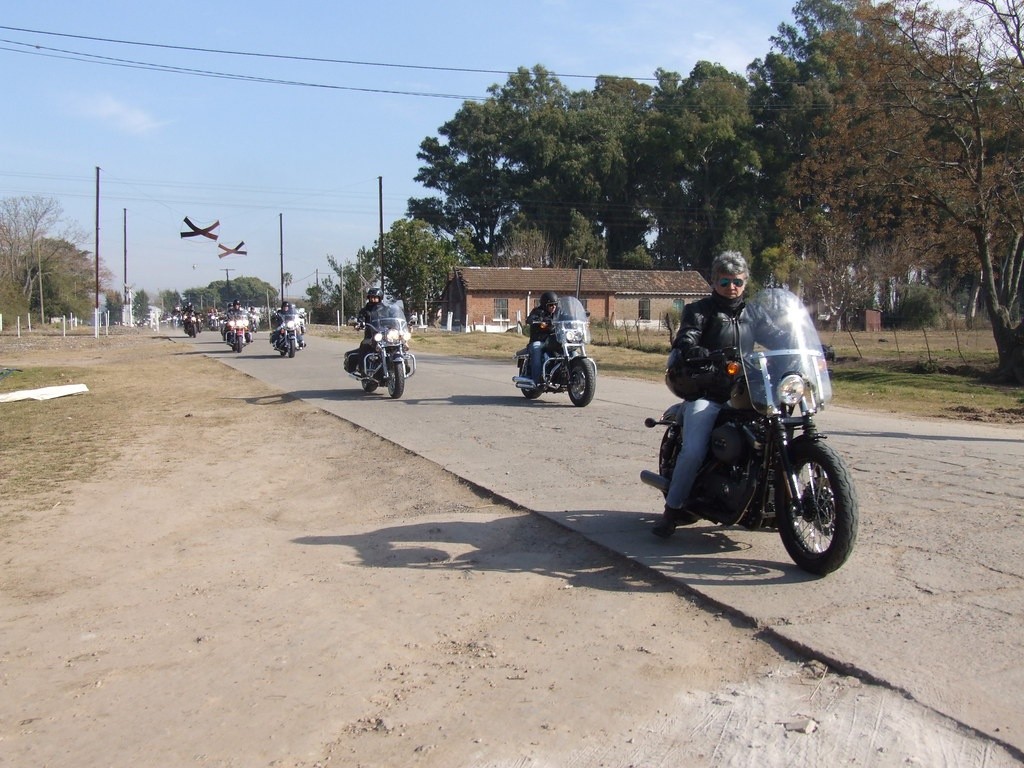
[651,252,837,546]
[226,299,255,343]
[171,307,180,316]
[526,291,559,386]
[276,302,306,347]
[184,302,192,316]
[354,288,410,377]
[208,308,215,317]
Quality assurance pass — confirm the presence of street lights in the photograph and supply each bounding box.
[574,257,588,299]
[220,268,235,303]
[123,282,134,330]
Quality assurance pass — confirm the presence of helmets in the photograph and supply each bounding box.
[367,288,385,299]
[228,302,233,308]
[250,307,254,311]
[540,291,560,310]
[281,301,289,306]
[187,302,192,309]
[233,300,240,306]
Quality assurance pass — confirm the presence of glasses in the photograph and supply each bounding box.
[550,303,559,307]
[714,278,748,286]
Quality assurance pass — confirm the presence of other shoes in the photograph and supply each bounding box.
[299,342,306,348]
[651,509,678,537]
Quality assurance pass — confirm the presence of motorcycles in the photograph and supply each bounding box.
[641,290,859,575]
[269,311,304,358]
[137,311,182,329]
[182,311,205,338]
[220,312,260,352]
[208,312,219,331]
[512,295,597,408]
[344,303,417,398]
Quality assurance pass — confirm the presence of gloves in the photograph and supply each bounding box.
[691,346,709,359]
[815,344,836,362]
